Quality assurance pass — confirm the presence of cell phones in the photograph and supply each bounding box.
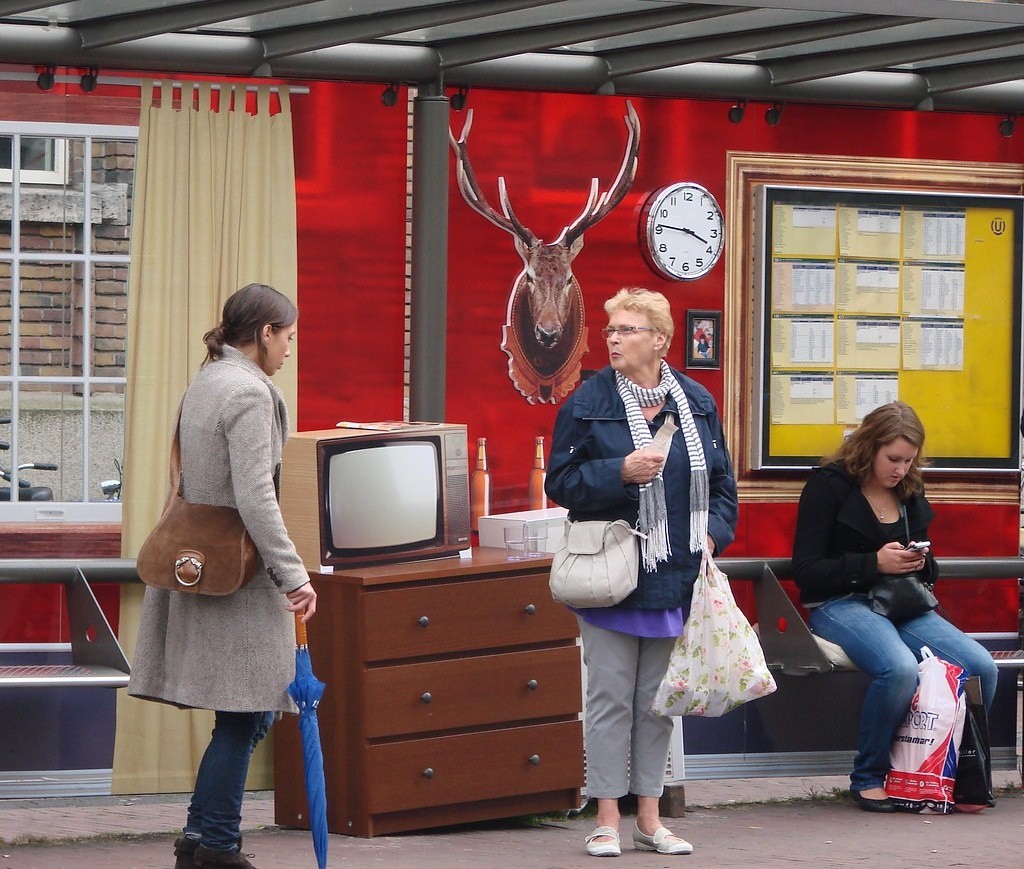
[905,542,932,551]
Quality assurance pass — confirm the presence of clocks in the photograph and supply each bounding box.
[640,182,725,283]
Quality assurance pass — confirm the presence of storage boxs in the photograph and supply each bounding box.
[478,507,569,553]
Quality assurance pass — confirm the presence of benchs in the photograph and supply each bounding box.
[0,569,132,688]
[753,565,1024,676]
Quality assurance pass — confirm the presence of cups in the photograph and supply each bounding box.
[524,522,548,558]
[505,525,529,560]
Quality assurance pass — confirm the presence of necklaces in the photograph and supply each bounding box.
[868,494,889,519]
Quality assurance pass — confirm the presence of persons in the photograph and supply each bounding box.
[696,334,710,358]
[126,283,319,869]
[790,401,998,812]
[544,284,741,856]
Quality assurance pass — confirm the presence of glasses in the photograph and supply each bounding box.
[600,324,660,339]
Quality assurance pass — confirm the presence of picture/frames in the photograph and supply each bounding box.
[686,310,722,370]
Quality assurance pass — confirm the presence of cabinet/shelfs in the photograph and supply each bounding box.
[274,548,584,838]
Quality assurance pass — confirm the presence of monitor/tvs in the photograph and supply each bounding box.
[276,420,472,574]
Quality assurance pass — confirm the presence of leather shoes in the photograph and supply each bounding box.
[849,786,895,814]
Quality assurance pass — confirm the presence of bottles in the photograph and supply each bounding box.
[470,437,493,534]
[528,436,547,512]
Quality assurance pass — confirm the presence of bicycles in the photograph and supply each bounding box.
[0,418,123,503]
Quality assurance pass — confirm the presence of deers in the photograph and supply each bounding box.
[447,99,641,404]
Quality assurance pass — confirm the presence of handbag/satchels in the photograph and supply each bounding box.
[883,646,967,815]
[650,549,778,717]
[549,516,648,610]
[134,496,266,597]
[868,570,939,620]
[951,702,996,813]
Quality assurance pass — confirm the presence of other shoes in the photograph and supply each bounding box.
[586,825,622,856]
[631,820,694,854]
[193,844,257,869]
[173,838,200,869]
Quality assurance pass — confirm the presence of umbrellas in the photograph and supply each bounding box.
[284,595,329,869]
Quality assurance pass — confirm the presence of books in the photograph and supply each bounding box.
[335,420,440,431]
